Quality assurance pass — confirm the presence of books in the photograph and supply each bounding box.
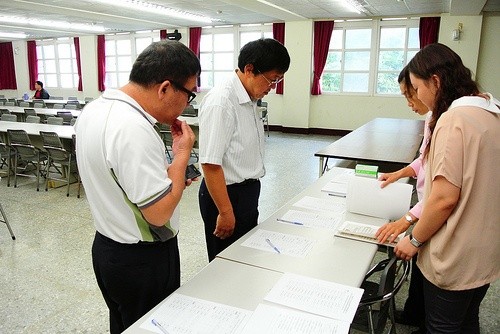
[334,175,413,250]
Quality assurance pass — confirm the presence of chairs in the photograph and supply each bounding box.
[0,95,411,334]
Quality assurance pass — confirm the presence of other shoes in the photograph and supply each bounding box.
[388,308,406,326]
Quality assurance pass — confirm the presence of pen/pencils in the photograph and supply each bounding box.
[151,319,168,333]
[277,218,304,225]
[265,238,280,254]
[328,193,346,198]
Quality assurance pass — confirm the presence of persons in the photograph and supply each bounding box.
[75,38,202,334]
[198,39,291,265]
[31,81,50,99]
[375,44,500,334]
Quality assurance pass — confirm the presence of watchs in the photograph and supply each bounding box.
[405,214,414,225]
[409,234,424,248]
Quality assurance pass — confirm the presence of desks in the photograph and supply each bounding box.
[0,99,426,334]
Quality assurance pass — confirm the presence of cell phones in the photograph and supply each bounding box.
[184,164,201,183]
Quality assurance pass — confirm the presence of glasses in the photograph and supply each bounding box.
[254,66,284,87]
[171,80,196,103]
